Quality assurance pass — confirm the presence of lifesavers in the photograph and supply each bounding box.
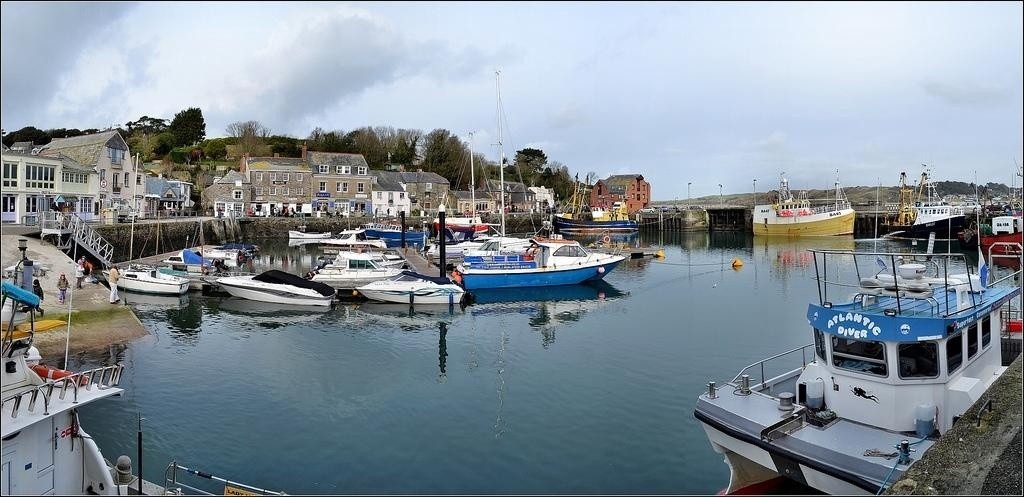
[603,235,610,242]
[30,363,88,385]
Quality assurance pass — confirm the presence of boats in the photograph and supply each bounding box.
[216,269,337,308]
[753,169,1019,269]
[104,242,259,296]
[2,282,290,497]
[693,246,1018,494]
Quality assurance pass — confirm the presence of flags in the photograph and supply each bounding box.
[980,249,988,290]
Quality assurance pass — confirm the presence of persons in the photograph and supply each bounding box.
[32,279,44,317]
[270,206,297,218]
[26,332,40,365]
[108,265,120,304]
[80,255,92,278]
[56,272,70,304]
[74,259,85,290]
